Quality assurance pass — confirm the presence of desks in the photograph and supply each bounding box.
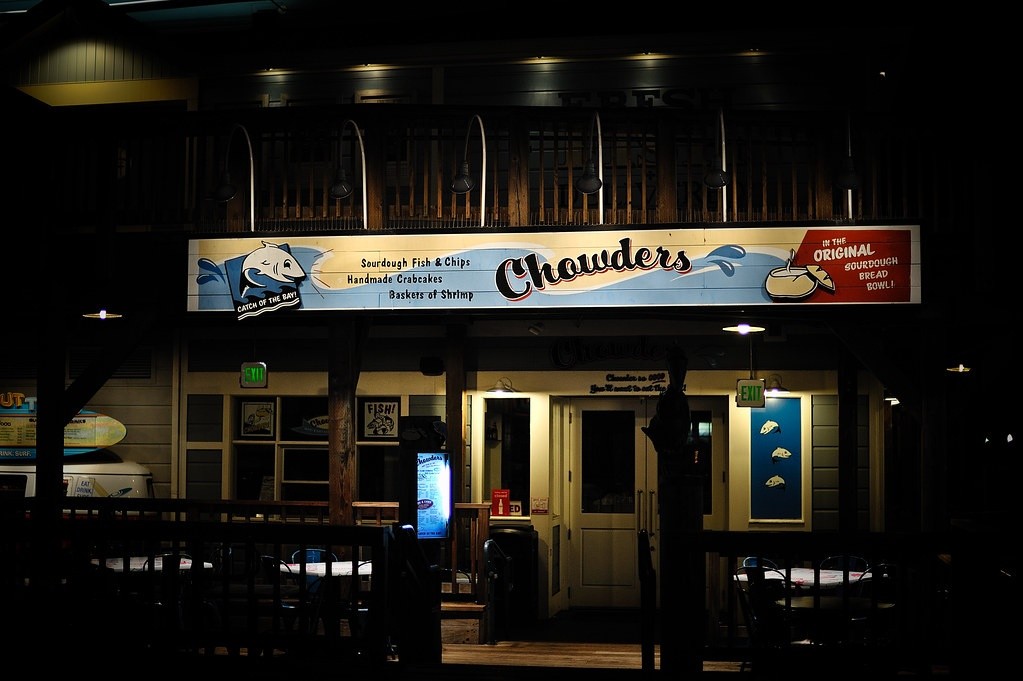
[731,567,891,663]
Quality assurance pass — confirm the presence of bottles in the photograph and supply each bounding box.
[498,499,503,515]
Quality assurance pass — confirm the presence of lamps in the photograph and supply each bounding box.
[216,123,256,232]
[703,107,728,223]
[449,114,487,227]
[489,379,517,395]
[528,321,545,336]
[329,119,367,229]
[766,379,790,392]
[420,356,443,376]
[575,110,605,224]
[836,111,859,221]
[722,320,766,334]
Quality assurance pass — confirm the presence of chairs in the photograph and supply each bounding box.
[732,550,899,672]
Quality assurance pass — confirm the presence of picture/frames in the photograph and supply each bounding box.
[235,398,276,443]
[358,397,401,441]
